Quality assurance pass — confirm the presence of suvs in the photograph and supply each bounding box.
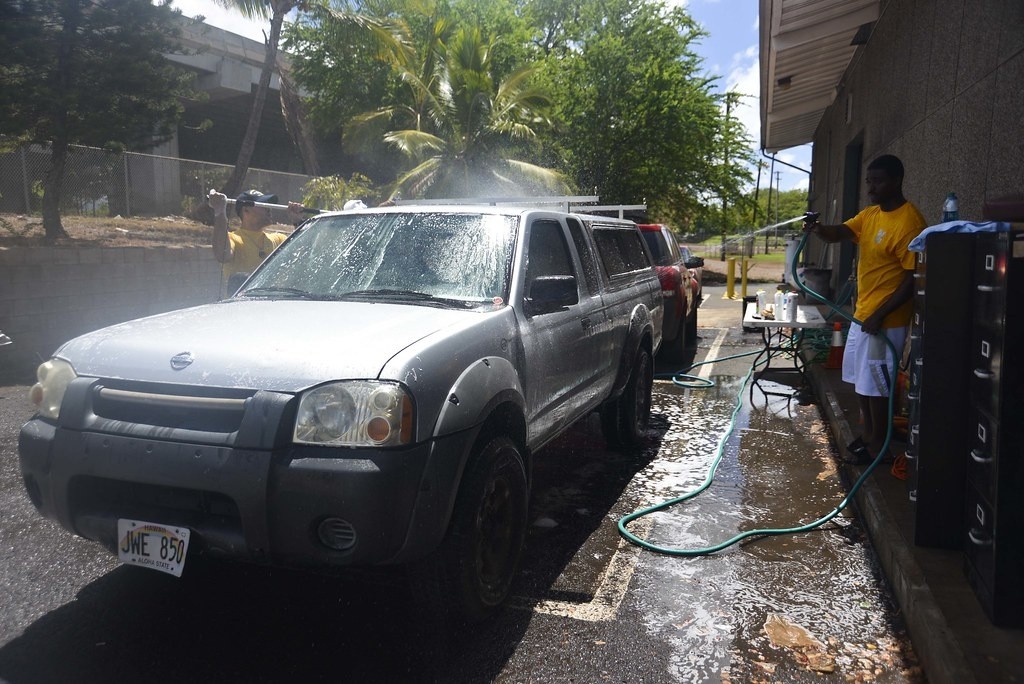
[628,223,696,368]
[17,206,668,644]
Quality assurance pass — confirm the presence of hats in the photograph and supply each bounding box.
[235,189,278,216]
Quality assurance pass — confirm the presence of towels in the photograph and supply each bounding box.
[907,218,1005,253]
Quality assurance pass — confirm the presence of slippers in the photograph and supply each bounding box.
[841,445,894,465]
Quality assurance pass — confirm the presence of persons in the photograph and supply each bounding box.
[342,198,396,212]
[205,187,308,305]
[800,155,931,468]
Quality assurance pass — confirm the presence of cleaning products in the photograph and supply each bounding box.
[941,190,958,225]
[755,288,769,316]
[785,288,799,323]
[946,196,961,222]
[774,286,784,318]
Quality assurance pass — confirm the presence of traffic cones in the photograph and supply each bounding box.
[820,321,844,368]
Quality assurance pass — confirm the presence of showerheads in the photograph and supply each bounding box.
[802,210,821,228]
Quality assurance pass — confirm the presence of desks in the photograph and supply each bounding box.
[742,302,828,386]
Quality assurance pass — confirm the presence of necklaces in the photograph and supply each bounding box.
[245,233,267,258]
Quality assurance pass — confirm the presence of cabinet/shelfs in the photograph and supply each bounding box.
[904,233,1024,629]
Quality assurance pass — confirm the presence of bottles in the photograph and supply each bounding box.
[941,192,959,223]
